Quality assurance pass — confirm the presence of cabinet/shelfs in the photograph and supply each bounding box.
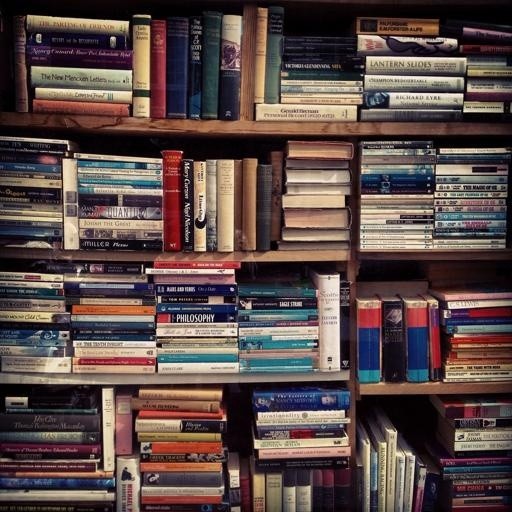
[0,0,512,511]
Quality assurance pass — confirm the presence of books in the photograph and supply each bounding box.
[357,279,511,384]
[355,397,512,511]
[358,142,510,251]
[1,1,512,123]
[1,384,355,511]
[1,140,353,253]
[1,263,350,373]
